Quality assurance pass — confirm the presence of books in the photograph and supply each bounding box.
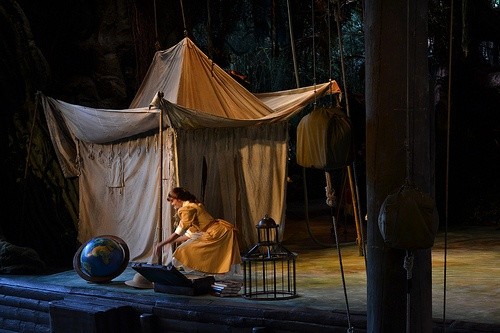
[211,280,243,297]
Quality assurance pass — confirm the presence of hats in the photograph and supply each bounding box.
[125,273,154,289]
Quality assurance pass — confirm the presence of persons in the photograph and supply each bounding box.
[155,187,242,281]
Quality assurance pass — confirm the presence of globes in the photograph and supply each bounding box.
[72,234,128,283]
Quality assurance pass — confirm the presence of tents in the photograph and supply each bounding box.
[24,37,364,266]
